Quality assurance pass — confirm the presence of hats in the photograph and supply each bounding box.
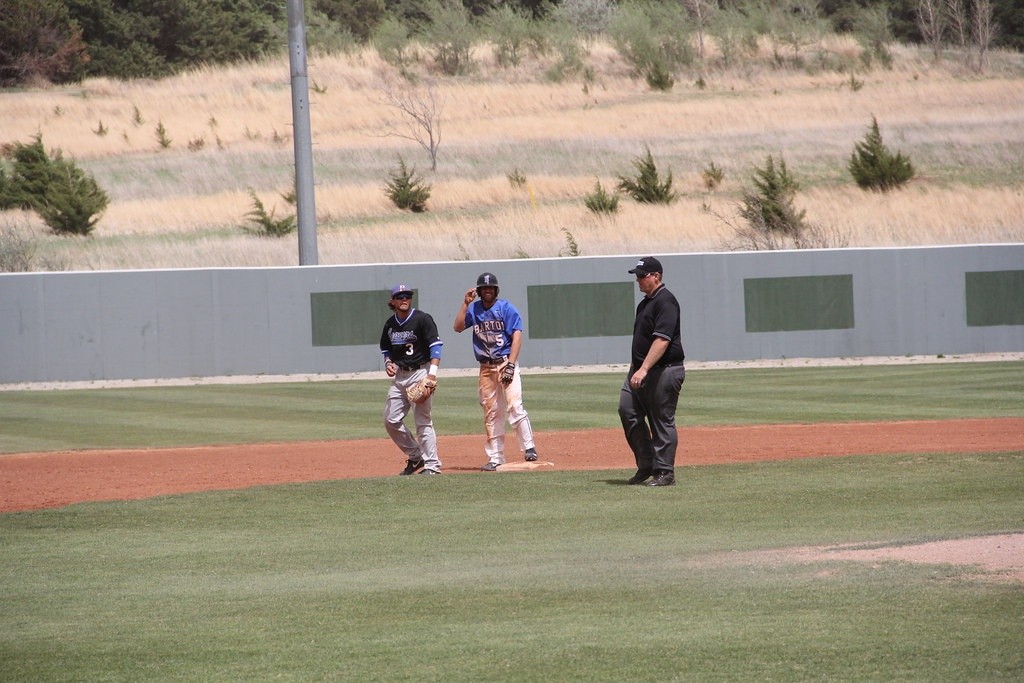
[391,284,413,296]
[628,257,662,275]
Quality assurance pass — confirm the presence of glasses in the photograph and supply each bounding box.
[393,293,412,300]
[637,272,656,279]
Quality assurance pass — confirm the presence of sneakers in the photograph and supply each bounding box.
[400,458,425,475]
[629,468,653,483]
[648,471,676,486]
[481,462,501,471]
[418,468,440,475]
[524,448,537,461]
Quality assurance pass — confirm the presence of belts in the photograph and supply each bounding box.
[398,365,420,372]
[652,361,683,368]
[480,357,504,365]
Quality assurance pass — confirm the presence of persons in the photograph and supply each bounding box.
[453,272,538,470]
[379,286,443,475]
[617,256,686,485]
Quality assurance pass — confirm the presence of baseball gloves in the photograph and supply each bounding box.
[405,378,438,404]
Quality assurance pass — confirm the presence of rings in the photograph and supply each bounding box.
[635,381,637,384]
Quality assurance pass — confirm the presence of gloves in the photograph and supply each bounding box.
[498,363,516,385]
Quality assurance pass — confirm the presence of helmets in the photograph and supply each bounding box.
[476,273,498,297]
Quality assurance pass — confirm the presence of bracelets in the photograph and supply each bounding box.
[385,359,391,367]
[428,364,438,377]
